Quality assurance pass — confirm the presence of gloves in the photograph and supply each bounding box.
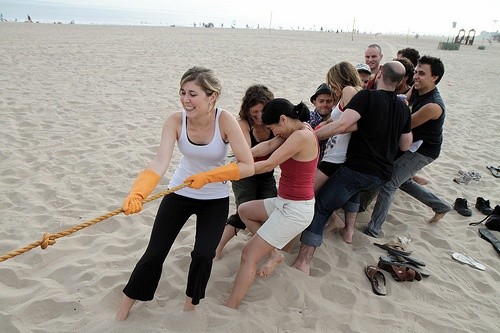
[122,169,162,215]
[182,162,240,190]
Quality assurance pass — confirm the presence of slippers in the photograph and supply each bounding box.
[452,252,487,271]
[453,164,500,184]
[364,242,431,296]
[479,227,500,254]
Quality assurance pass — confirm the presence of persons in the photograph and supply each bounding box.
[291,60,413,277]
[389,57,415,94]
[397,47,420,86]
[116,66,255,322]
[225,98,320,308]
[281,61,365,254]
[26,14,32,22]
[354,62,372,89]
[306,82,335,129]
[216,84,277,257]
[365,43,383,89]
[362,55,452,238]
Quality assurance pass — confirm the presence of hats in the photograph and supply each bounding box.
[355,63,372,75]
[309,83,338,104]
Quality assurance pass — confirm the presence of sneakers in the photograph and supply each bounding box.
[453,198,473,217]
[475,197,493,215]
